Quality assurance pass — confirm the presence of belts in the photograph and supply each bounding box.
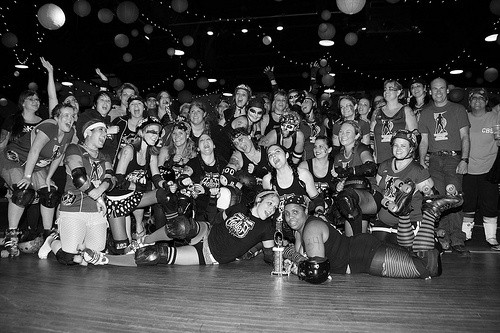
[433,151,462,156]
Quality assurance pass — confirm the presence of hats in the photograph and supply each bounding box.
[284,196,307,210]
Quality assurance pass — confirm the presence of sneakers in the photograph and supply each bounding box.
[452,245,470,256]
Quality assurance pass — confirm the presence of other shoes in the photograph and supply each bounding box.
[484,238,500,250]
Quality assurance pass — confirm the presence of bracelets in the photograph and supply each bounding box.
[23,174,32,178]
[101,80,109,88]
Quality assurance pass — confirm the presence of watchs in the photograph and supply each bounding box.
[461,157,469,164]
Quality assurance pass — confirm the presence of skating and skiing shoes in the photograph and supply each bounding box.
[381,177,416,217]
[74,242,109,266]
[4,228,21,258]
[421,184,463,220]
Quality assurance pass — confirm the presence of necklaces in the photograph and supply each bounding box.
[208,160,215,166]
[83,144,99,154]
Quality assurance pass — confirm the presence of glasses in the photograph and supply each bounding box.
[289,94,300,99]
[248,109,263,114]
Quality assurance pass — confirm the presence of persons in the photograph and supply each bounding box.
[74,188,280,267]
[283,178,463,284]
[39,57,85,142]
[0,77,500,253]
[96,68,140,124]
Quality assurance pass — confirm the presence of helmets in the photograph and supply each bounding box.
[82,119,106,139]
[246,98,266,114]
[298,255,330,284]
[235,84,252,98]
[390,129,417,147]
[279,112,300,127]
[135,116,162,139]
[468,88,488,100]
[171,121,191,139]
[304,94,317,107]
